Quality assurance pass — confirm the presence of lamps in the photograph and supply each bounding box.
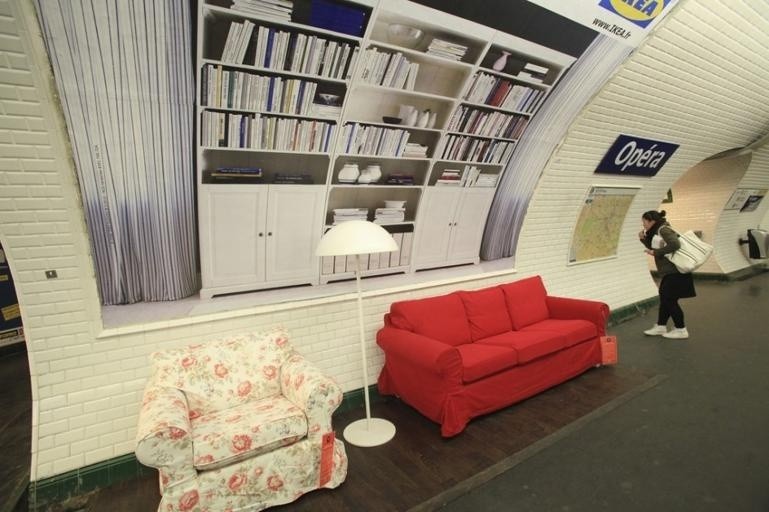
[313,219,400,448]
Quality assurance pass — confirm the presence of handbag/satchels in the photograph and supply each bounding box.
[662,229,714,274]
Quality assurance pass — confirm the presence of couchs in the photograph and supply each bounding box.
[376,275,610,439]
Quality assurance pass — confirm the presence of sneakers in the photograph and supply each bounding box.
[663,327,689,339]
[644,324,668,336]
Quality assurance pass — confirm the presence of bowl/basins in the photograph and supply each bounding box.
[382,116,401,125]
[318,93,341,105]
[383,199,409,210]
[386,23,425,50]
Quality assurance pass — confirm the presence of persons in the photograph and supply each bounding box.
[638,210,697,339]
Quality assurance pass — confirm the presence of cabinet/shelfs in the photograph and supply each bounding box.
[197,0,577,302]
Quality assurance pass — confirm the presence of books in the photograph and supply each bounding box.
[222,19,360,81]
[359,47,420,91]
[435,165,499,187]
[212,168,315,184]
[333,209,405,222]
[343,123,428,158]
[427,39,468,61]
[464,73,544,113]
[441,135,515,164]
[201,65,342,120]
[231,0,293,22]
[202,110,337,154]
[449,104,528,140]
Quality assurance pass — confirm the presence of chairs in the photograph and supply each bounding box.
[135,327,348,512]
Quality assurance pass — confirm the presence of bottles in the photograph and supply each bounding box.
[399,103,438,130]
[492,50,512,71]
[336,159,382,185]
